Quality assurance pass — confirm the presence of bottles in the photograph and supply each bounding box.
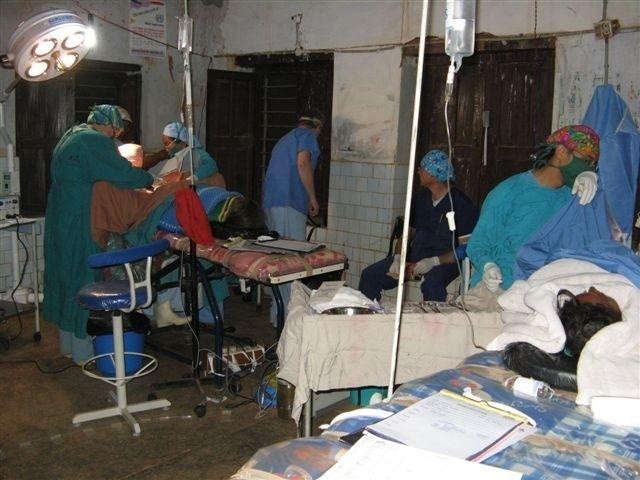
[503,374,549,402]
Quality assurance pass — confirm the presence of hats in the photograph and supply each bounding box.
[421,150,455,182]
[87,104,132,129]
[547,124,600,156]
[163,123,188,144]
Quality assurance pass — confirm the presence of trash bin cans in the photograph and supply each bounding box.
[86,309,152,377]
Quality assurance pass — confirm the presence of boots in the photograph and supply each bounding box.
[155,300,191,328]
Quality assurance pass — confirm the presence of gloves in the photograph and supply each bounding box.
[413,256,440,275]
[389,254,400,280]
[571,170,598,206]
[482,263,503,293]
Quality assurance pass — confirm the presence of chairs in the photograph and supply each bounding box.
[70,234,178,440]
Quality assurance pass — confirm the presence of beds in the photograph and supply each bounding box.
[273,279,522,438]
[133,213,348,388]
[227,331,640,480]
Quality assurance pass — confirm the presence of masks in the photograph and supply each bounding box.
[555,143,593,188]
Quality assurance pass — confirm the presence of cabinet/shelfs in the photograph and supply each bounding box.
[1,216,47,347]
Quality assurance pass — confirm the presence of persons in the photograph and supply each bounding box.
[359,148,477,302]
[464,124,639,381]
[260,106,326,328]
[40,102,266,373]
